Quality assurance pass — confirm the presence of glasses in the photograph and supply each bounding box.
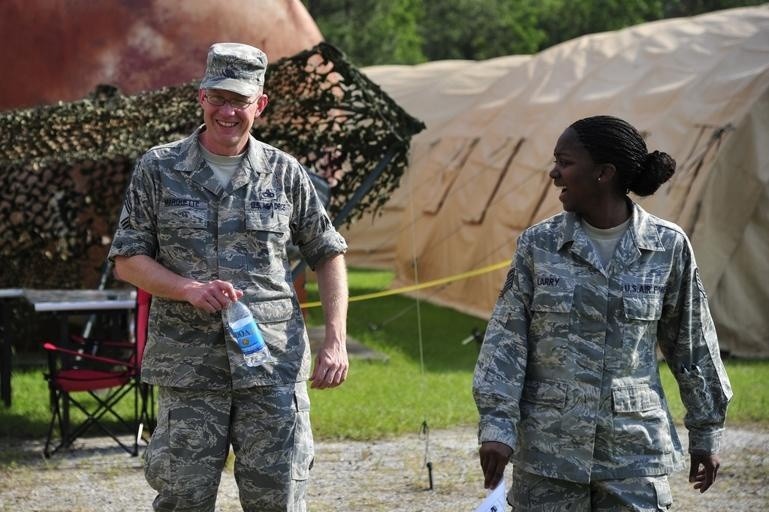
[201,92,262,110]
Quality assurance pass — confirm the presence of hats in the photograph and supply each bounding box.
[197,41,270,97]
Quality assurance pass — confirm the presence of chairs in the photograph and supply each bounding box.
[41,288,152,460]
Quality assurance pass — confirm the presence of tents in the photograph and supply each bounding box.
[306,4,769,361]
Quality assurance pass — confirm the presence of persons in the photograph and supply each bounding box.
[107,39,349,511]
[471,114,732,512]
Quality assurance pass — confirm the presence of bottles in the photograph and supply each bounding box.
[225,299,271,367]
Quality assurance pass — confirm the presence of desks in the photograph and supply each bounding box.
[0,289,143,448]
